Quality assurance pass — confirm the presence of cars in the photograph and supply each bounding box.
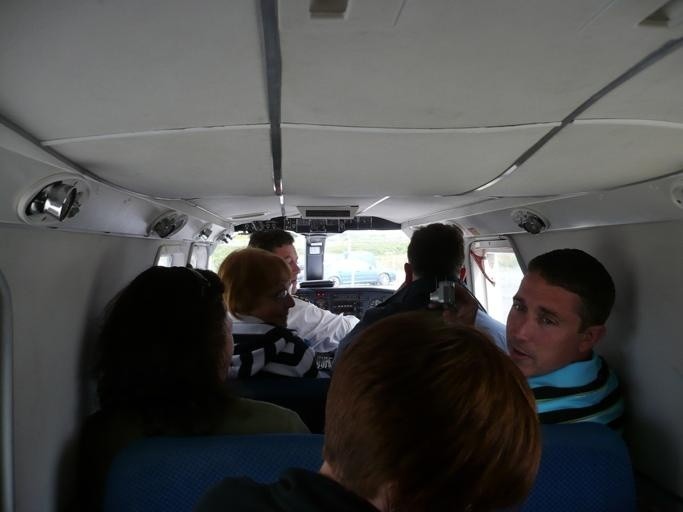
[324,249,395,286]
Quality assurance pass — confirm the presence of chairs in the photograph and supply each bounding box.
[520,421,638,510]
[111,433,324,511]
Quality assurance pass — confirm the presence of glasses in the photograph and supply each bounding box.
[270,288,288,300]
[177,266,211,296]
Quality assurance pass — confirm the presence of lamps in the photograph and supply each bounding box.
[155,218,176,238]
[28,181,78,222]
[516,214,544,234]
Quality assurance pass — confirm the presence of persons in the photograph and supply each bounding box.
[217,249,319,382]
[73,263,312,512]
[248,229,362,353]
[194,310,544,512]
[333,222,508,357]
[506,250,632,444]
